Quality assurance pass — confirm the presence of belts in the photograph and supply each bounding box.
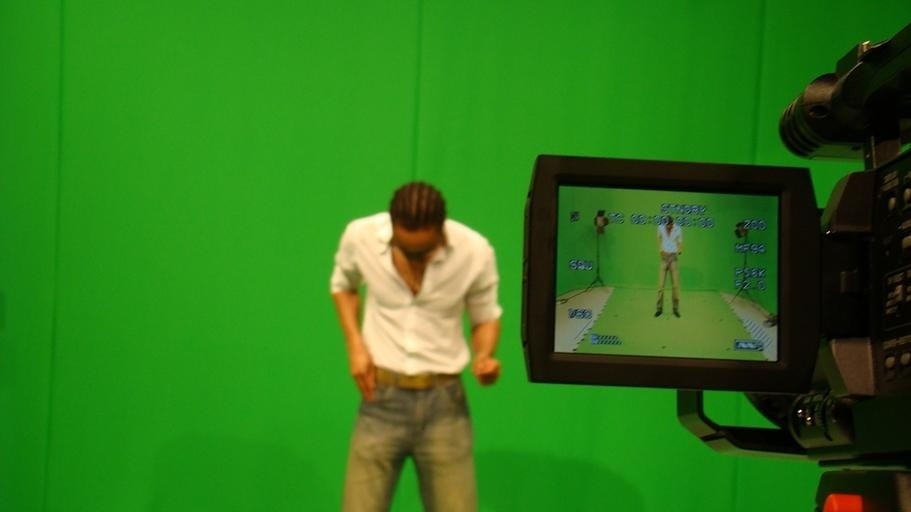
[373,369,457,389]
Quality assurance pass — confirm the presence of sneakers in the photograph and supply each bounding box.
[674,312,679,317]
[655,312,663,317]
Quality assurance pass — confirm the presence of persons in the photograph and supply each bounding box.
[653,215,683,318]
[329,181,501,512]
